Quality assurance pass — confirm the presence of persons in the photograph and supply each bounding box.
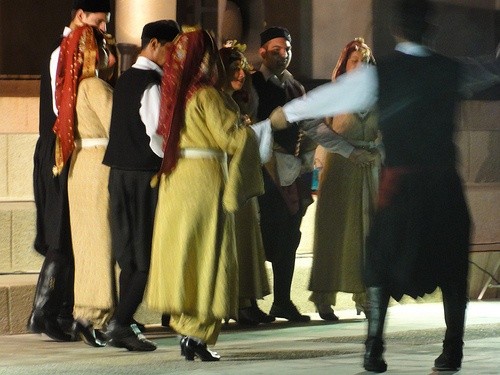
[134,28,287,362]
[26,0,500,373]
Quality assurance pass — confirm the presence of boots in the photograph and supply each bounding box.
[434,340,464,369]
[363,337,387,373]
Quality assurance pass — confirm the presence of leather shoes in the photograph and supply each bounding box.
[26,309,72,342]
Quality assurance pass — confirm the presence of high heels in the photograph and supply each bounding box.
[353,299,369,319]
[308,298,339,321]
[74,319,107,347]
[180,336,221,361]
[269,300,311,322]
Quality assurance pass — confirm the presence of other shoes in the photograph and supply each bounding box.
[236,302,275,323]
[109,323,157,351]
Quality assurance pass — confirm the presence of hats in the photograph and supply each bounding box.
[73,0,114,12]
[261,27,292,46]
[142,19,181,42]
[219,47,247,70]
